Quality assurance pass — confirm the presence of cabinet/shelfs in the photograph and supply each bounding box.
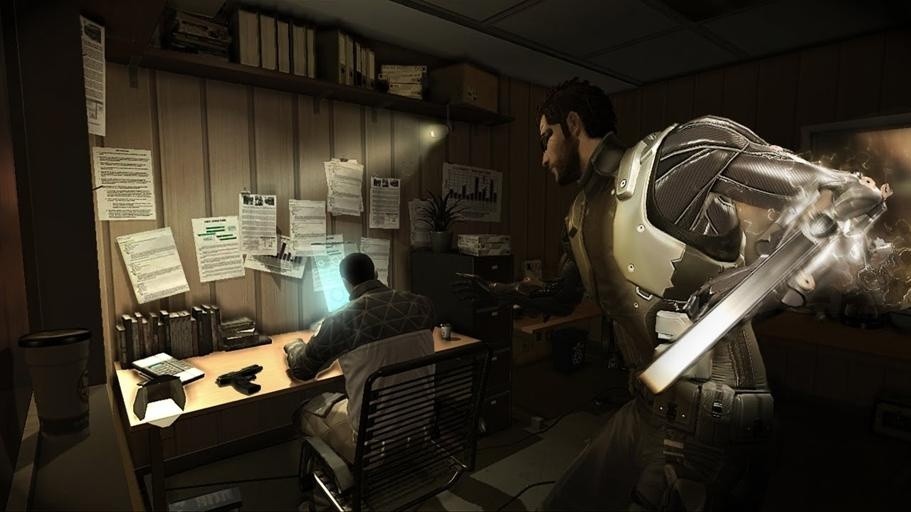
[410,250,514,434]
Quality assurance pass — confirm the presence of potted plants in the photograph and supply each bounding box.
[411,187,472,250]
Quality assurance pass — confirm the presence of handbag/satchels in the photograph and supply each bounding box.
[652,380,773,455]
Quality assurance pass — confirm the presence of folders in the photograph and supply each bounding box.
[315,30,376,91]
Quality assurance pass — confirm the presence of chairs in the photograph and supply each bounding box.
[295,341,493,512]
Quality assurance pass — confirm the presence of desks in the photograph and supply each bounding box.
[506,301,609,429]
[114,321,479,512]
